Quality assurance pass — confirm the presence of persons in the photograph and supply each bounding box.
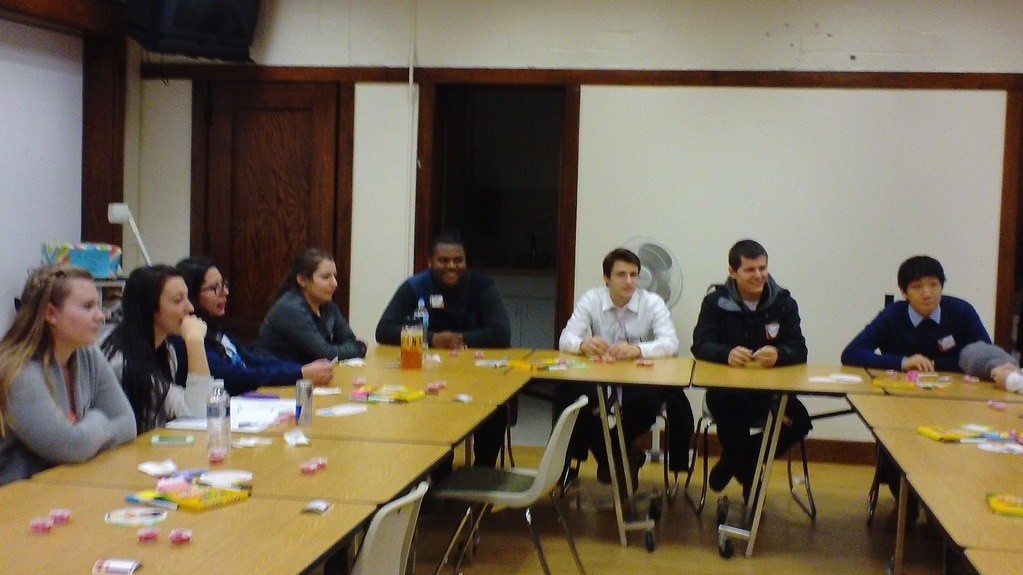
[557,247,681,484]
[840,255,992,536]
[252,248,369,366]
[990,362,1023,395]
[375,228,520,480]
[690,239,813,514]
[94,264,217,435]
[175,259,334,397]
[0,264,137,489]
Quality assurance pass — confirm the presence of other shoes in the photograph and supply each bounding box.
[709,455,733,493]
[598,448,647,500]
[743,466,766,508]
[924,517,943,545]
[886,500,918,534]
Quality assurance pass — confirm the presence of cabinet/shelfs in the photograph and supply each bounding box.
[479,274,559,348]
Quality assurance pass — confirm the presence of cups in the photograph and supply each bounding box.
[296,381,313,426]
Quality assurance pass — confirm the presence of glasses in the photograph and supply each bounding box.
[201,278,229,297]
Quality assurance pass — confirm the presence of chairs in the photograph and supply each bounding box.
[559,390,679,501]
[431,394,590,574]
[349,478,431,574]
[686,393,816,520]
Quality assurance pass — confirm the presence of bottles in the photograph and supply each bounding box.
[414,299,429,361]
[400,317,423,368]
[207,379,230,453]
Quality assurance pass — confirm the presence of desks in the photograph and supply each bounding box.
[0,341,1023,574]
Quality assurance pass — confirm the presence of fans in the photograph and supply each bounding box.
[622,236,683,308]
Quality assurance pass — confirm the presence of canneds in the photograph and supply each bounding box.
[295,379,313,426]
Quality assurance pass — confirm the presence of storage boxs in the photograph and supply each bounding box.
[40,243,122,279]
